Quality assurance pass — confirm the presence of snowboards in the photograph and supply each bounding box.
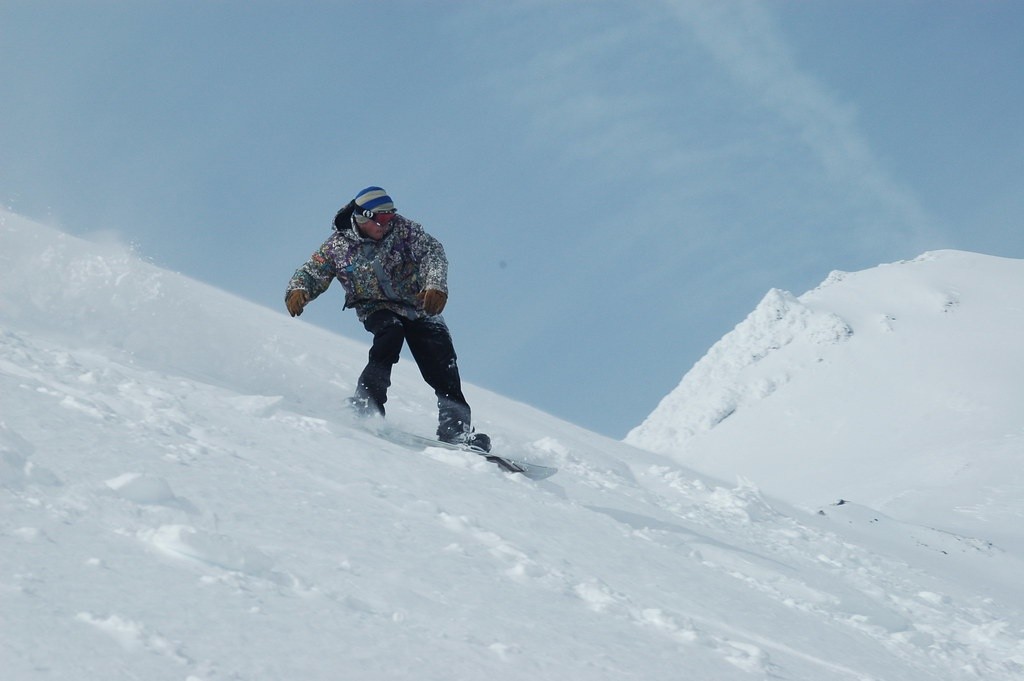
[272,394,558,481]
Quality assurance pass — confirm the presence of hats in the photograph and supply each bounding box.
[353,186,394,223]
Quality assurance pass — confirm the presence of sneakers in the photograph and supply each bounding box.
[438,420,492,453]
[343,392,382,417]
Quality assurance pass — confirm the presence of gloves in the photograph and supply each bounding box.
[285,289,310,317]
[416,289,448,315]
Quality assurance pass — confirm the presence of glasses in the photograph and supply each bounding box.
[371,208,397,226]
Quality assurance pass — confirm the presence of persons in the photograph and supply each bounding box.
[284,186,493,454]
